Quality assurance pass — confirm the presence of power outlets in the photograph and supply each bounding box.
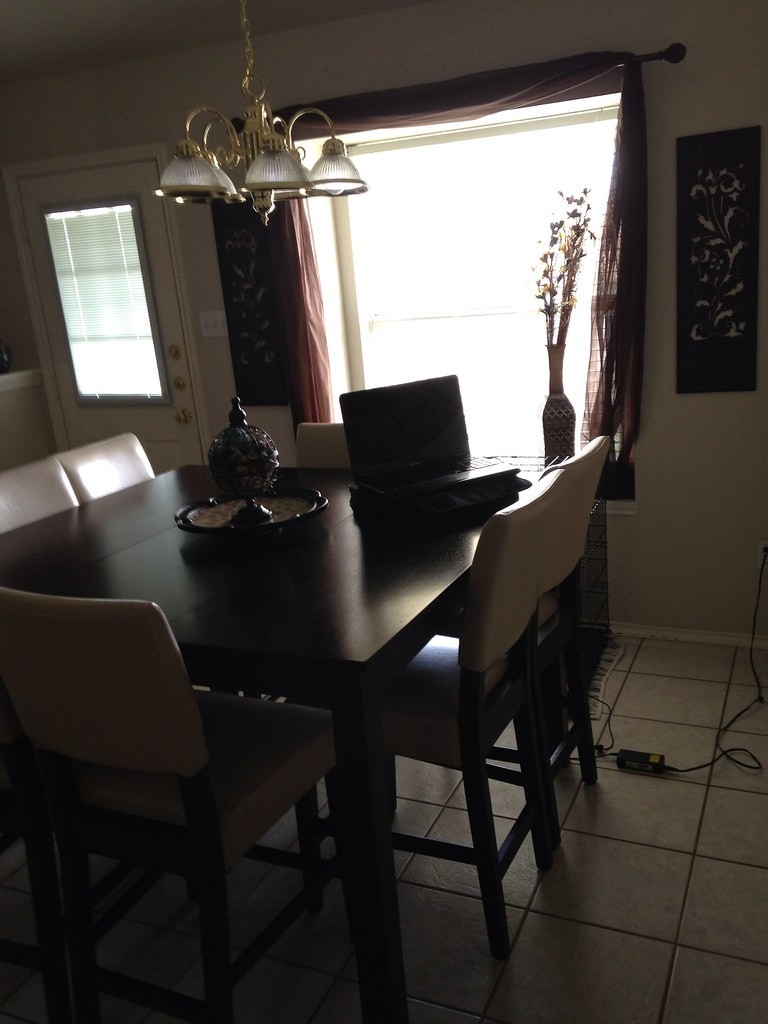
[756,540,768,570]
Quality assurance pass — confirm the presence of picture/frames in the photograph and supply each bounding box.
[210,197,289,407]
[674,125,761,394]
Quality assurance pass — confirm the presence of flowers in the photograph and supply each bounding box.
[531,188,598,345]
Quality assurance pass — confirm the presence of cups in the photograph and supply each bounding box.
[0,339,12,372]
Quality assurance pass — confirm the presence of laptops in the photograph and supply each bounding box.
[341,376,520,499]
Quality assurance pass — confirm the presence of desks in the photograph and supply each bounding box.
[0,460,540,1024]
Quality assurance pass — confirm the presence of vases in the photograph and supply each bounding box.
[542,344,576,466]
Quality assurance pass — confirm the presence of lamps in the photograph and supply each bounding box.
[150,0,372,228]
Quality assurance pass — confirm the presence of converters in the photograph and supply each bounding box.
[616,749,665,774]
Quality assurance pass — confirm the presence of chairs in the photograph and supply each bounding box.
[0,456,80,534]
[440,435,615,847]
[55,431,156,506]
[296,422,351,467]
[0,695,141,1024]
[0,586,338,1024]
[274,476,555,959]
